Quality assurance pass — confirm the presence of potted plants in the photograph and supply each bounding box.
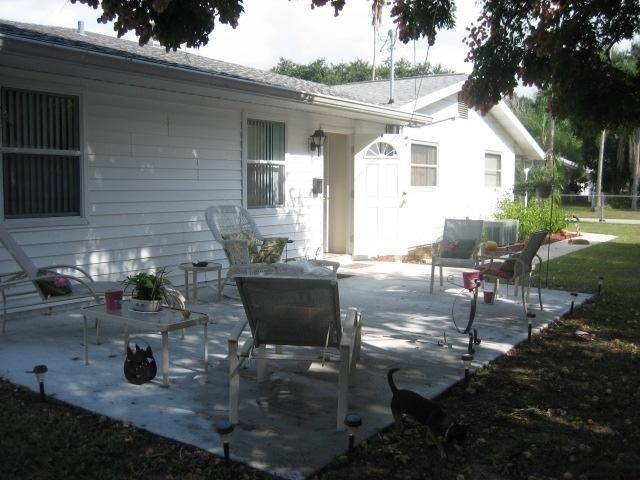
[118,264,188,313]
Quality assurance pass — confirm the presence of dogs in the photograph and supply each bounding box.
[386,367,470,471]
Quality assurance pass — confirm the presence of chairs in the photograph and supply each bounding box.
[427,217,550,315]
[207,201,363,434]
[0,223,109,345]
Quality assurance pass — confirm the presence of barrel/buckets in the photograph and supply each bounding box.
[463,269,480,290]
[105,288,123,311]
[483,290,494,303]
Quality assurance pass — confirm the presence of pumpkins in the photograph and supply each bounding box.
[485,242,497,251]
[560,229,569,236]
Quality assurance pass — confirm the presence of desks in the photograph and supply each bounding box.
[83,300,207,388]
[180,261,222,303]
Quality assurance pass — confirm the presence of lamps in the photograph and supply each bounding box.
[310,129,327,157]
